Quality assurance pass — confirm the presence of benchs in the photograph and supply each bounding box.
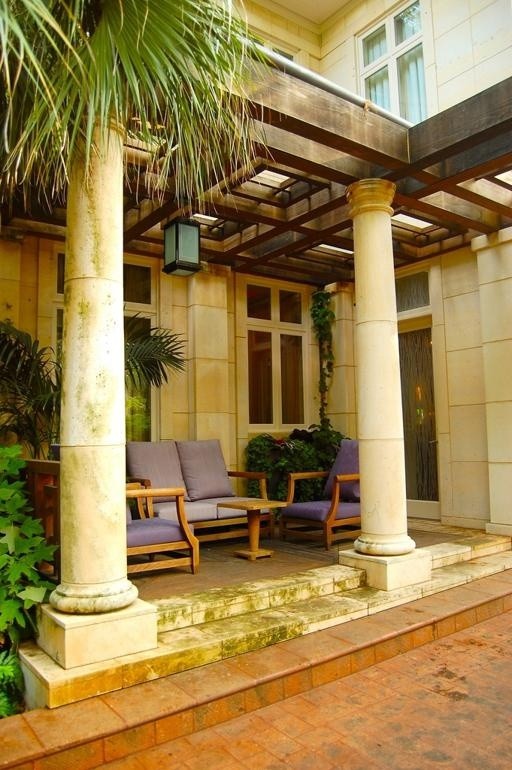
[126,441,276,547]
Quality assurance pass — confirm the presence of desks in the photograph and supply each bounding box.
[216,500,292,561]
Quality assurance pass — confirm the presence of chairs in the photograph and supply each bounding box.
[278,440,360,551]
[126,476,199,579]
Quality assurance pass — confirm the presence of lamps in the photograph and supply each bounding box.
[162,215,202,277]
